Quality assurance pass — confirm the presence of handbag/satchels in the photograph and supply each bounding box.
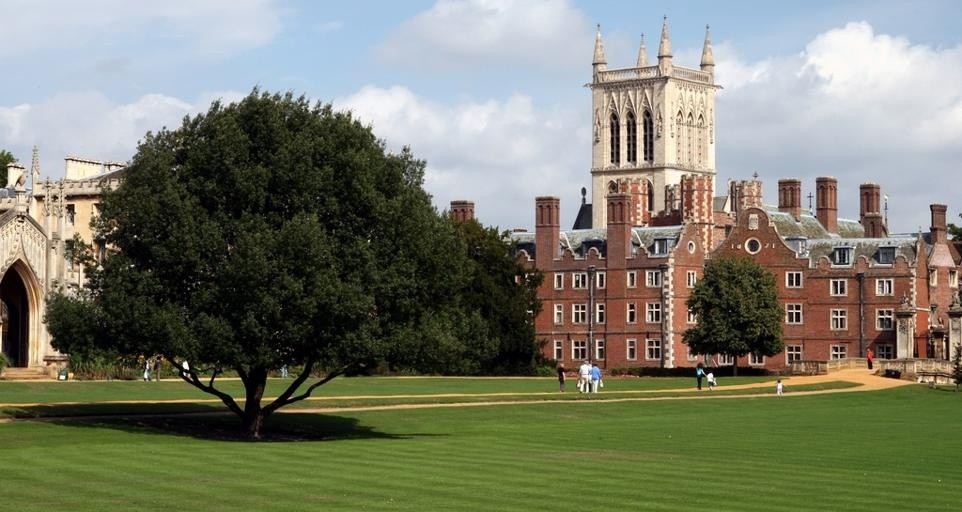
[599,379,603,389]
[576,380,581,388]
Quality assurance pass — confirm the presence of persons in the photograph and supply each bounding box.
[776,380,782,401]
[282,365,288,378]
[181,360,192,378]
[142,356,162,382]
[705,370,714,391]
[557,363,565,392]
[866,347,874,369]
[695,362,705,390]
[576,358,602,393]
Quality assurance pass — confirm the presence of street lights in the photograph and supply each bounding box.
[929,304,937,358]
[856,272,865,358]
[658,263,669,368]
[587,264,596,364]
